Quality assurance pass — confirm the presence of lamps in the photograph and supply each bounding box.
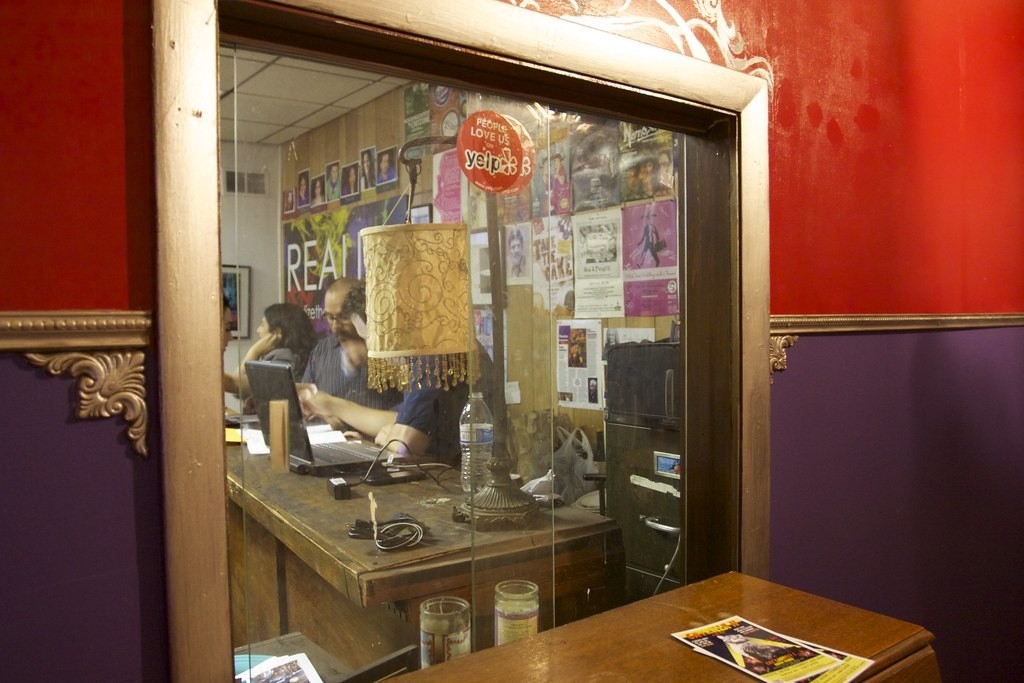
[357,135,540,532]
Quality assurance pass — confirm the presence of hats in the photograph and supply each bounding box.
[641,210,657,219]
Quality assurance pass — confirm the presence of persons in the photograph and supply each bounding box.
[285,150,397,214]
[574,177,619,212]
[221,278,519,476]
[474,305,492,341]
[590,379,597,402]
[624,156,672,200]
[637,217,660,269]
[603,327,620,360]
[569,332,586,367]
[542,153,569,216]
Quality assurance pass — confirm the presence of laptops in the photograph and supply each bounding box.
[245,360,406,477]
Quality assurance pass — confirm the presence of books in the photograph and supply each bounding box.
[306,424,347,444]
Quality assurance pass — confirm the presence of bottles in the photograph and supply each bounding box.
[458,392,493,491]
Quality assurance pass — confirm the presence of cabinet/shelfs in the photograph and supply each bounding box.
[596,339,680,611]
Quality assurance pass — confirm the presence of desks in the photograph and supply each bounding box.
[224,442,624,672]
[387,570,944,683]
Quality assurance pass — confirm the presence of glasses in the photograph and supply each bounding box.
[321,308,353,326]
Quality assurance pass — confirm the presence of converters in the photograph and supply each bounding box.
[327,478,351,499]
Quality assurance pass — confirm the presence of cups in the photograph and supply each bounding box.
[495,580,539,646]
[419,597,470,669]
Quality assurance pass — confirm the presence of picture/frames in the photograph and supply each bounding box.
[223,264,252,339]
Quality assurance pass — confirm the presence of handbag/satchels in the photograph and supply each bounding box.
[539,425,598,506]
[655,240,667,252]
